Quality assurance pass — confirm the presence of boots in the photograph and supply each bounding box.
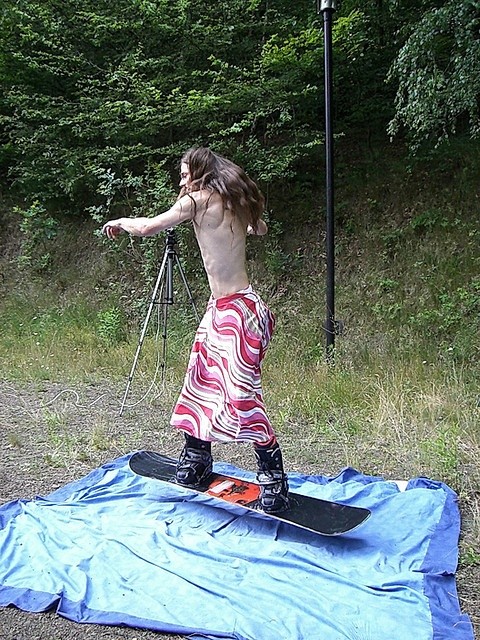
[175,431,212,485]
[253,443,289,510]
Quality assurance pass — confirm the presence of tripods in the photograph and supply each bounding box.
[120,228,202,415]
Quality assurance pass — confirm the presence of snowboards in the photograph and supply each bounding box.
[128,450,371,537]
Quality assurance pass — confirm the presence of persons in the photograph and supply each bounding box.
[100,142,293,516]
